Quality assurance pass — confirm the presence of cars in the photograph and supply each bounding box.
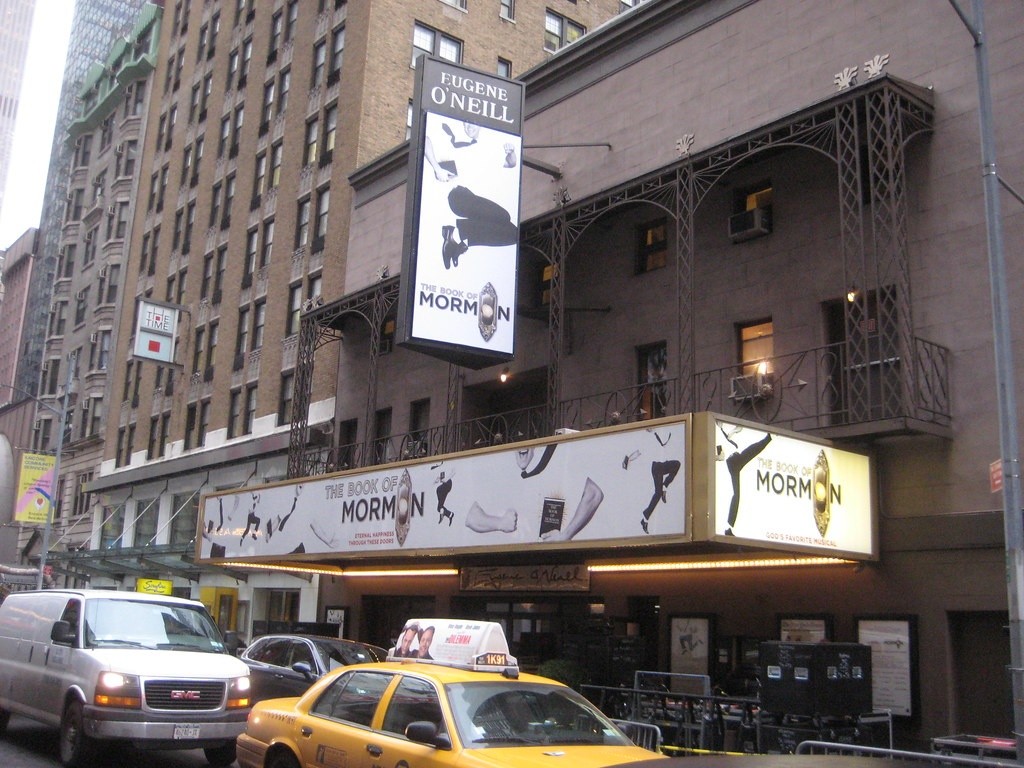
[236,616,670,767]
[239,629,388,711]
[601,751,980,768]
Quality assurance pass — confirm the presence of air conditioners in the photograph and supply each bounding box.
[407,441,426,456]
[731,373,774,402]
[379,339,391,355]
[727,208,770,242]
[29,33,145,439]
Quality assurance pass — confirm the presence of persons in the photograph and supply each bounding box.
[622,427,681,534]
[266,484,339,554]
[394,624,424,657]
[466,443,604,542]
[715,420,777,536]
[240,494,262,546]
[202,496,240,557]
[424,116,518,269]
[431,461,455,526]
[676,619,703,655]
[411,626,435,660]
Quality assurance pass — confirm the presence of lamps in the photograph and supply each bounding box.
[499,367,510,382]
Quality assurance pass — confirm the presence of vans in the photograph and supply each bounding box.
[0,589,252,767]
